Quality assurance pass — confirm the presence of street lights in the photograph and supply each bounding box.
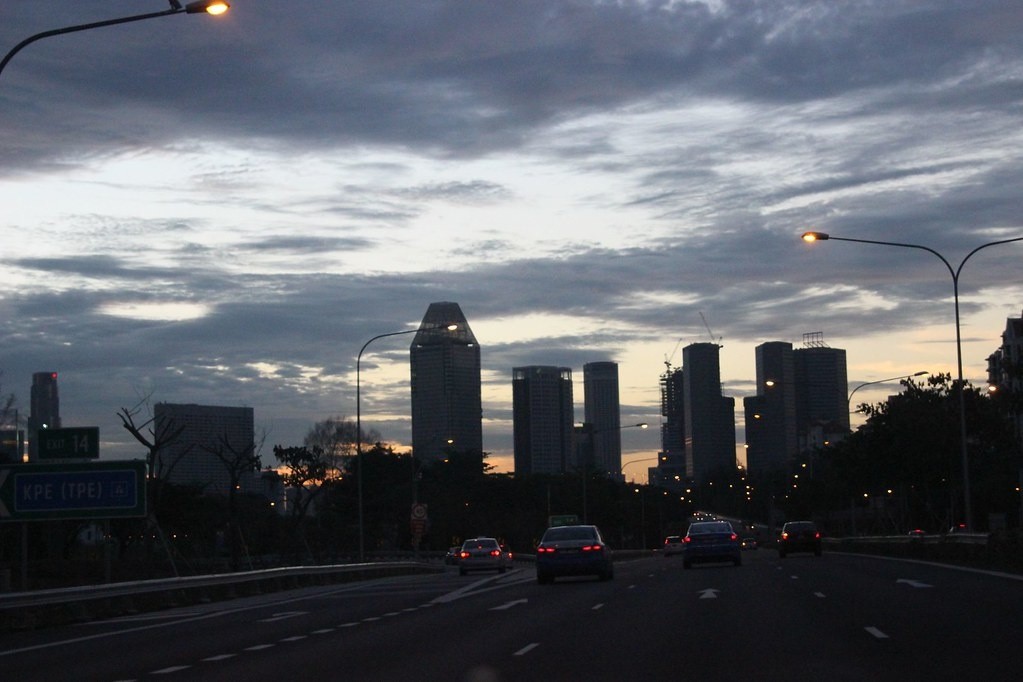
[801,229,1023,534]
[356,321,460,562]
[620,456,668,472]
[581,422,649,527]
[764,371,929,535]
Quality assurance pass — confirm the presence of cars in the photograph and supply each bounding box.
[908,529,926,536]
[741,538,757,550]
[499,543,514,569]
[779,521,822,559]
[445,546,462,566]
[949,522,968,534]
[682,522,741,570]
[664,535,685,557]
[458,538,507,576]
[536,524,613,585]
